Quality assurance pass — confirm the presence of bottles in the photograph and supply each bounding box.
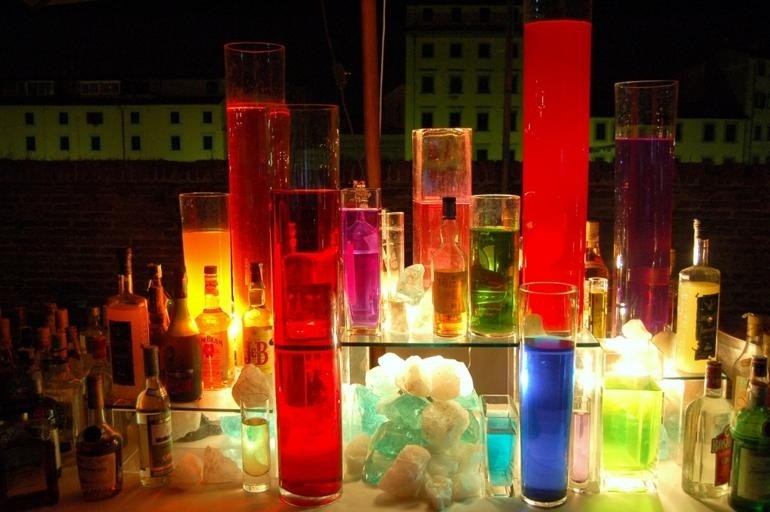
[679,307,769,512]
[431,195,469,340]
[238,392,276,494]
[676,217,722,374]
[158,262,202,402]
[569,319,668,492]
[0,245,173,511]
[194,264,237,394]
[135,344,176,490]
[240,257,274,380]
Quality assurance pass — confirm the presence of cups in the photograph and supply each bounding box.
[223,38,290,326]
[514,280,578,508]
[467,194,521,340]
[477,392,519,500]
[411,126,472,280]
[606,72,681,342]
[177,189,236,336]
[341,186,424,341]
[268,104,344,511]
[582,220,611,341]
